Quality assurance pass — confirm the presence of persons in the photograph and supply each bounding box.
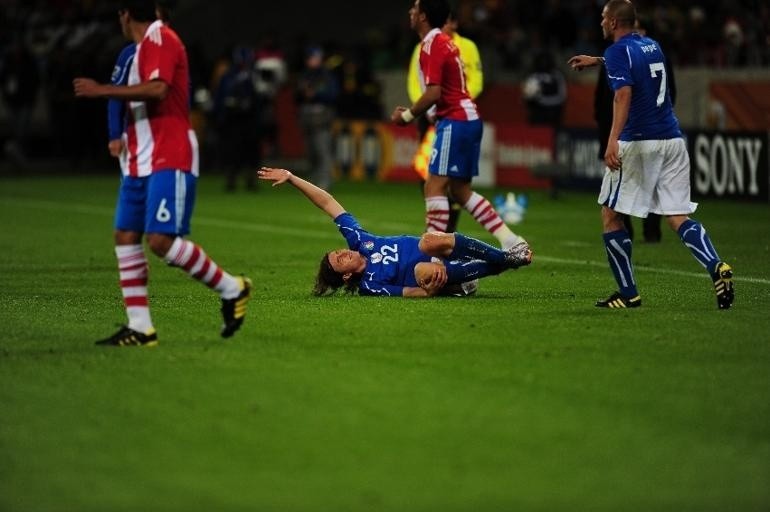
[74,1,255,345]
[254,162,536,298]
[387,0,529,263]
[566,0,736,308]
[404,3,484,231]
[591,15,679,243]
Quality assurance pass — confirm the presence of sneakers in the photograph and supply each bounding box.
[220,275,253,339]
[94,327,159,349]
[594,292,641,308]
[507,235,533,269]
[713,261,734,311]
